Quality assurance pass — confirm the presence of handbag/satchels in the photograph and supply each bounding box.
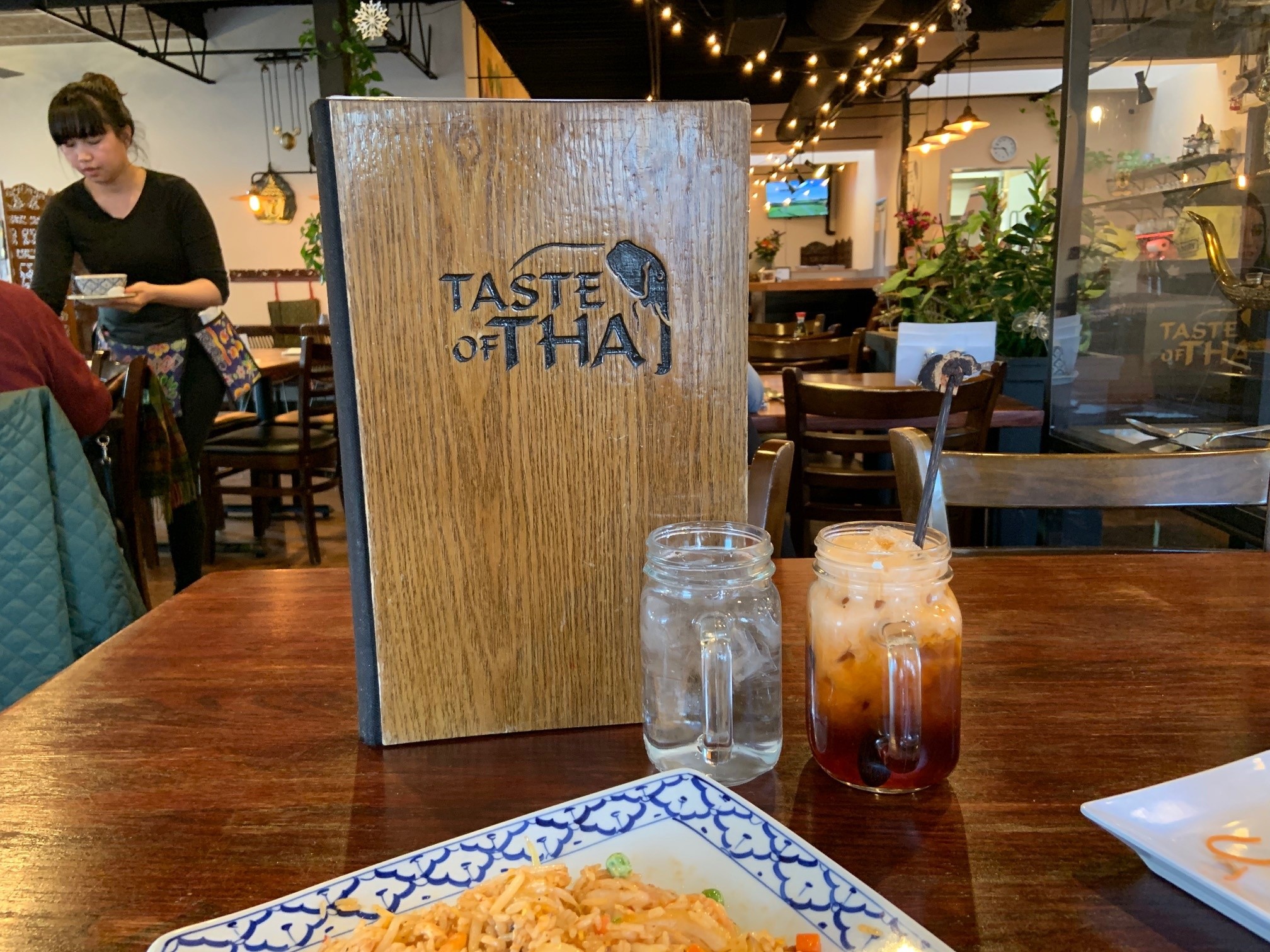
[90,455,115,523]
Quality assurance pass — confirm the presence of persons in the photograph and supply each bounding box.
[1107,192,1270,427]
[27,73,228,592]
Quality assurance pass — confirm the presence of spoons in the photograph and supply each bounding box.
[858,379,955,788]
[1162,423,1270,450]
[1126,416,1270,442]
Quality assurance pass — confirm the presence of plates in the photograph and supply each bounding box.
[1078,746,1270,942]
[143,764,969,952]
[66,292,137,309]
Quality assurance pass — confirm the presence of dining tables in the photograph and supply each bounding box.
[0,550,1269,951]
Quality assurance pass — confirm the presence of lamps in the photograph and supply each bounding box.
[908,34,992,155]
[1132,57,1156,107]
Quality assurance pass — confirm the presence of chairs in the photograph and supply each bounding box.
[778,365,1008,556]
[105,321,355,566]
[886,423,1270,554]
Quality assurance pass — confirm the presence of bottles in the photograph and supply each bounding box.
[793,311,808,338]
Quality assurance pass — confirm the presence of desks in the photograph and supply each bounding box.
[746,322,868,375]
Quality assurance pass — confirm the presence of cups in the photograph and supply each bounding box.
[804,520,961,794]
[638,517,786,789]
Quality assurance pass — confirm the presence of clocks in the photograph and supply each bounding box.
[990,135,1017,163]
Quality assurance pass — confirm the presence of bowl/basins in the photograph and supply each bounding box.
[75,272,128,296]
